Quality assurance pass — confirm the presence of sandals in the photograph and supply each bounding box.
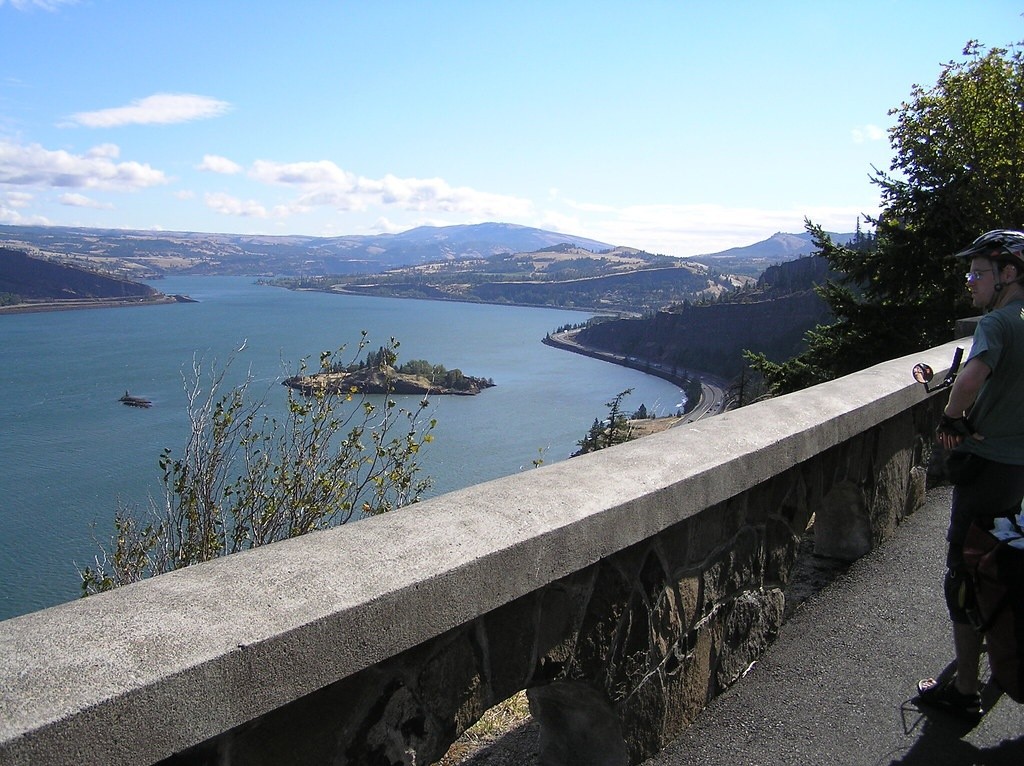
[917,678,982,721]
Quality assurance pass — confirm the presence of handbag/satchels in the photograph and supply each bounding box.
[963,515,1024,704]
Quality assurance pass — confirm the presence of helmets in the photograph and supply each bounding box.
[954,229,1024,269]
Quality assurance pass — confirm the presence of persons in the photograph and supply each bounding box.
[913,364,933,382]
[916,229,1024,716]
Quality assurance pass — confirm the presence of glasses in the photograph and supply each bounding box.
[965,269,992,279]
